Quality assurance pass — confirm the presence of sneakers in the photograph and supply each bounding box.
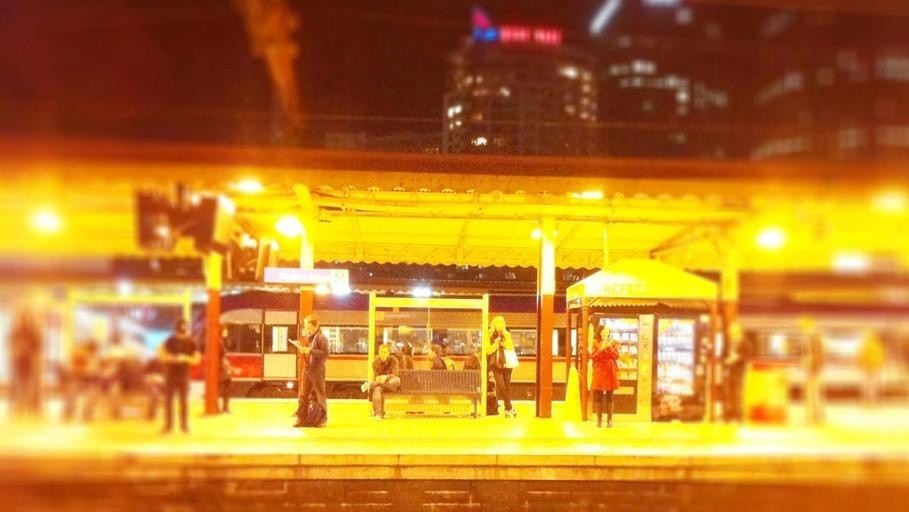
[360,382,371,392]
[505,409,517,419]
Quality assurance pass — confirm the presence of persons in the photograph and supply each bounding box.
[291,314,330,427]
[225,324,261,352]
[798,317,827,424]
[516,331,534,353]
[855,325,886,405]
[360,344,401,422]
[219,338,233,413]
[486,316,518,418]
[8,302,202,435]
[341,326,481,355]
[719,322,756,425]
[590,324,619,427]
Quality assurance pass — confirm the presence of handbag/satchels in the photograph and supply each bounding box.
[496,350,519,368]
[296,395,324,426]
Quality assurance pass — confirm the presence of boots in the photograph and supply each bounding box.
[595,402,613,427]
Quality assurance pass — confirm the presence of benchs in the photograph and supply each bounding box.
[381,369,482,418]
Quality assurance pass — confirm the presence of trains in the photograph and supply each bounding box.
[191,309,909,422]
